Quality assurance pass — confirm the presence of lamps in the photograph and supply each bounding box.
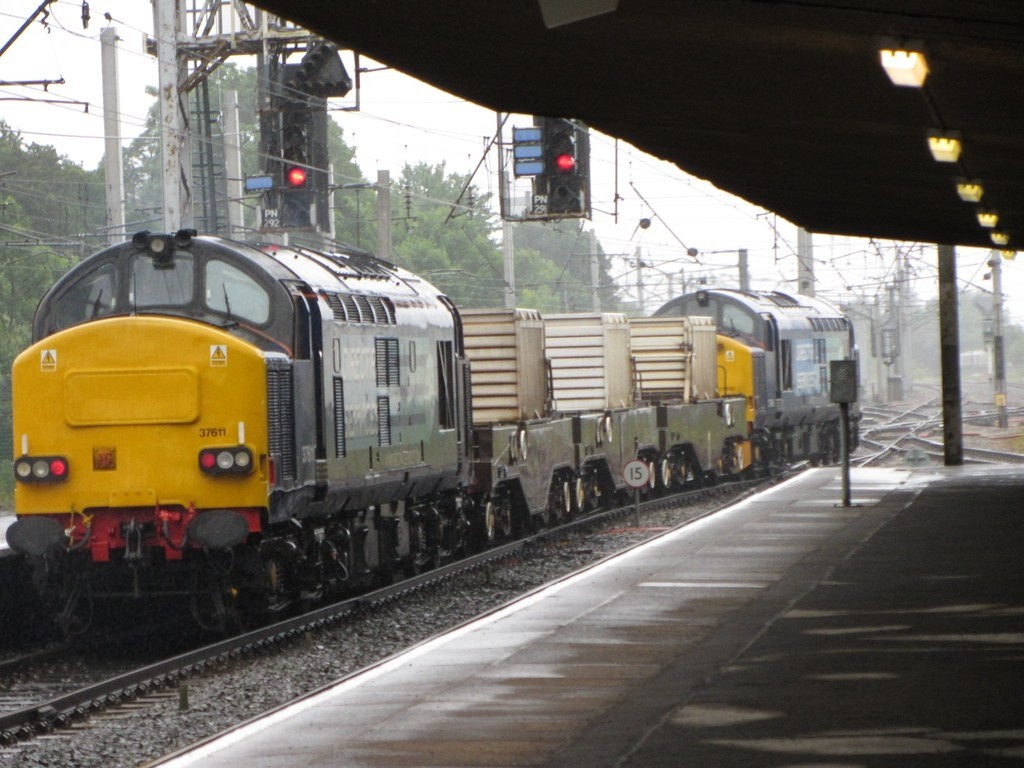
[953,177,1016,258]
[927,127,963,162]
[876,35,938,87]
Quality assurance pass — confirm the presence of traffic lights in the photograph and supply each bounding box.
[544,117,581,213]
[277,106,313,196]
[285,39,354,100]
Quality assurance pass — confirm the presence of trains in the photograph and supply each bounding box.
[1,216,867,646]
